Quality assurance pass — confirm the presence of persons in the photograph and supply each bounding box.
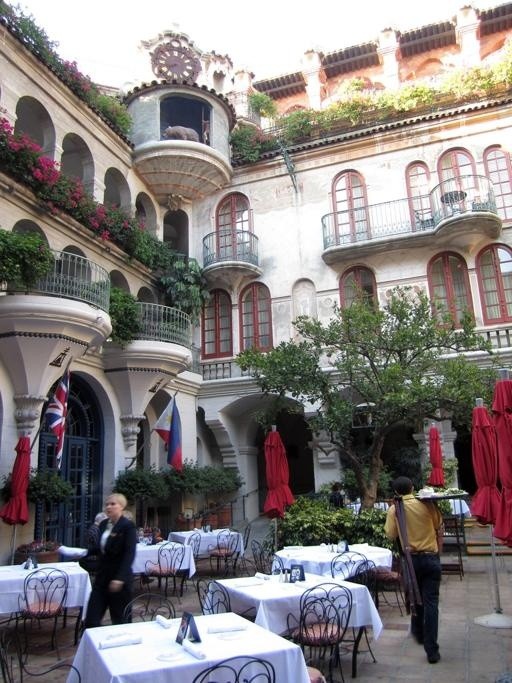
[86,512,108,555]
[327,480,352,509]
[83,492,138,645]
[134,523,165,586]
[382,476,447,663]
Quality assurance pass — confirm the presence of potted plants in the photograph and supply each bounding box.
[111,456,247,540]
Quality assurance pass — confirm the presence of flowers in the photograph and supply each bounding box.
[0,115,146,246]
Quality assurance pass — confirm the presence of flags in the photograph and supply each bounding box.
[43,364,72,470]
[156,394,186,473]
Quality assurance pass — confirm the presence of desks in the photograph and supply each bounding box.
[440,191,467,216]
[414,490,469,582]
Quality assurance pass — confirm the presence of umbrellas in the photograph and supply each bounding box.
[493,378,512,552]
[470,394,512,615]
[262,423,294,559]
[0,428,35,566]
[427,422,445,493]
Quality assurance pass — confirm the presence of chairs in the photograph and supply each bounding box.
[471,193,490,212]
[411,208,434,230]
